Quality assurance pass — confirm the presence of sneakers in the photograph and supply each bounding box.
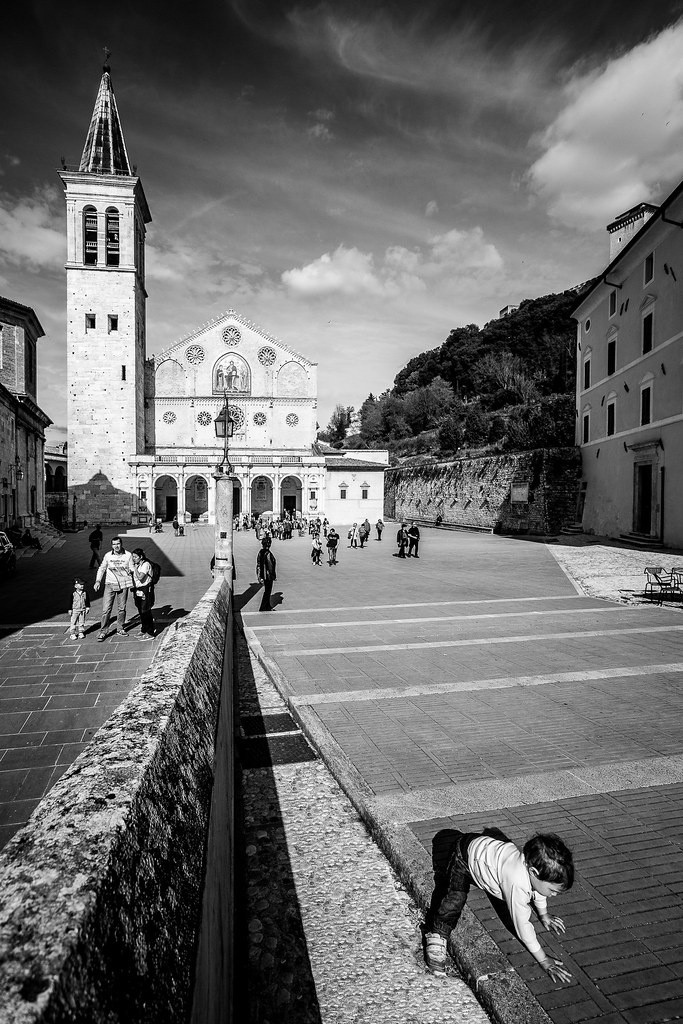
[133,632,144,638]
[421,929,449,972]
[116,629,129,637]
[97,633,106,642]
[140,633,155,641]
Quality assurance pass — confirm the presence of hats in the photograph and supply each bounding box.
[75,578,84,584]
[402,523,406,527]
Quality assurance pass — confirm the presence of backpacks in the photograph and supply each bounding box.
[142,558,161,584]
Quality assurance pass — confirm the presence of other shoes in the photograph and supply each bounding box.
[279,537,281,539]
[78,633,85,639]
[313,561,316,565]
[69,634,76,640]
[283,536,292,540]
[415,555,419,558]
[351,546,353,549]
[408,554,410,557]
[403,553,405,557]
[319,561,323,566]
[398,554,401,557]
[356,547,358,549]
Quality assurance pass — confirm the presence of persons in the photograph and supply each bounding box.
[173,518,184,537]
[348,519,370,549]
[68,577,90,640]
[326,529,339,565]
[257,537,276,612]
[5,525,43,550]
[149,518,163,533]
[426,832,574,983]
[235,508,329,540]
[375,519,385,541]
[93,537,155,642]
[311,533,322,566]
[89,523,103,569]
[397,522,420,559]
